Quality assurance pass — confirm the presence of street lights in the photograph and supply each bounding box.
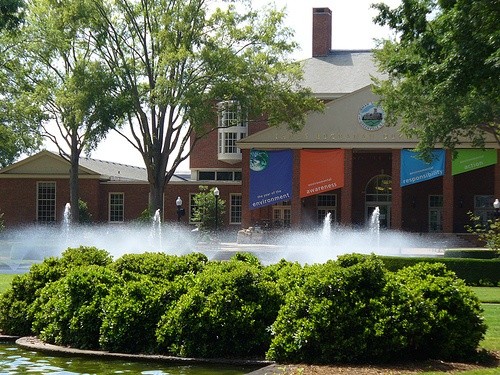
[175,196,186,222]
[213,187,219,230]
